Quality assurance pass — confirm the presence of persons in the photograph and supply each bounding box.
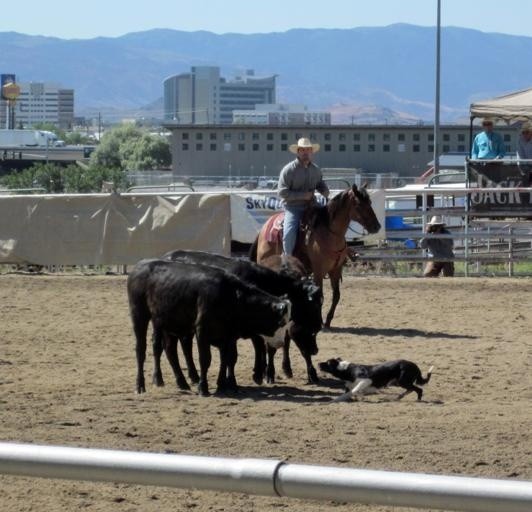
[278,138,330,256]
[420,216,455,277]
[471,118,506,220]
[516,122,532,220]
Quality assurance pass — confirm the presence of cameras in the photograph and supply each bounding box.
[429,226,436,233]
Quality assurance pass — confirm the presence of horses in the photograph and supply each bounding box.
[248,182,382,329]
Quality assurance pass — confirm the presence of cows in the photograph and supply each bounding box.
[160,248,320,392]
[126,258,292,397]
[258,255,320,384]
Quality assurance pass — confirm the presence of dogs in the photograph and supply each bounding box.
[318,357,434,403]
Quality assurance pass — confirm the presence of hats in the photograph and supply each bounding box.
[426,215,445,225]
[289,137,320,155]
[521,122,532,132]
[480,117,496,127]
